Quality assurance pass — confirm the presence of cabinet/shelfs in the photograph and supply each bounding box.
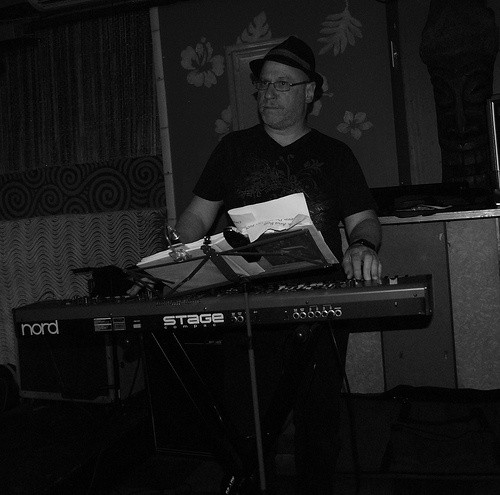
[338,207,500,395]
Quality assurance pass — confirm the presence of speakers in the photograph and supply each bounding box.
[141,331,232,458]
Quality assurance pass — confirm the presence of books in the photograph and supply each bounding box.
[136,232,338,291]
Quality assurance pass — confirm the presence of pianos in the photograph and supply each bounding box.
[11,272,431,330]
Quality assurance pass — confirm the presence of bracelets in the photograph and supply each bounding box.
[350,239,375,250]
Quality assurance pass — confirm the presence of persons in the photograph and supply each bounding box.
[127,35,383,495]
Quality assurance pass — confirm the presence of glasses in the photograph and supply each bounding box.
[252,78,313,94]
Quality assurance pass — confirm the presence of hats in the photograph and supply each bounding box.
[250,35,324,96]
